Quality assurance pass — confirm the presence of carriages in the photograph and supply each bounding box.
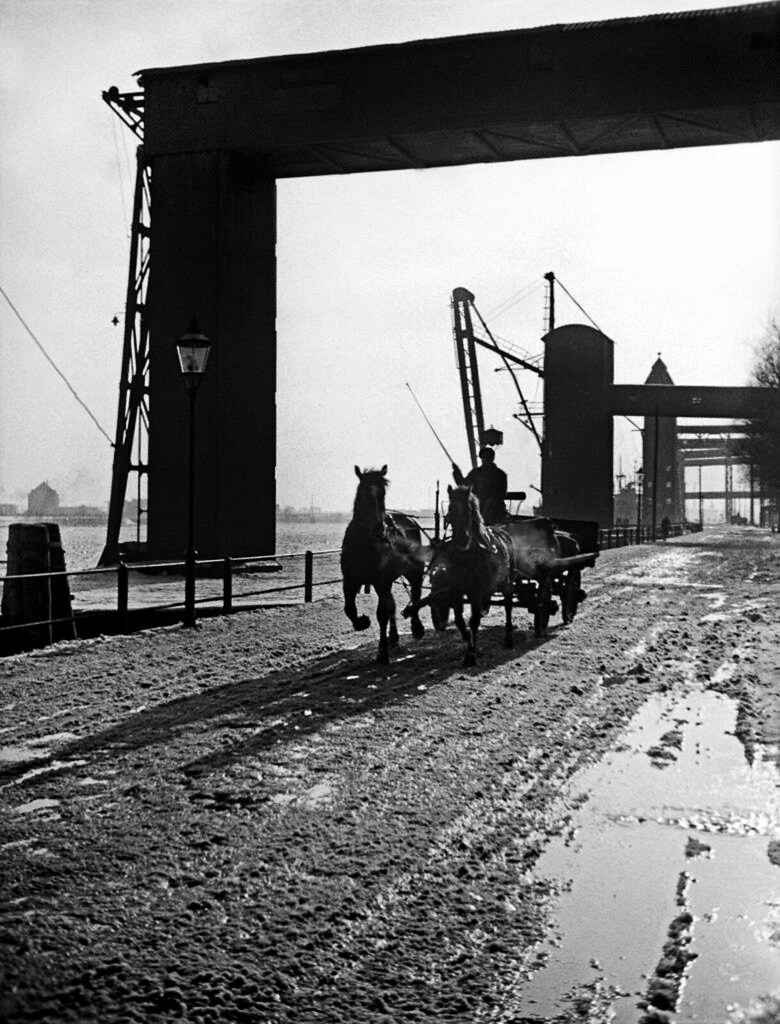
[335,464,600,669]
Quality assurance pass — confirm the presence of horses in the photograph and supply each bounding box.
[339,463,425,666]
[444,484,516,669]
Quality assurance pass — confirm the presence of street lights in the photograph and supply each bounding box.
[633,467,647,545]
[175,327,213,626]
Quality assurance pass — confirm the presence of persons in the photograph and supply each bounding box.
[452,448,508,525]
[661,517,670,540]
